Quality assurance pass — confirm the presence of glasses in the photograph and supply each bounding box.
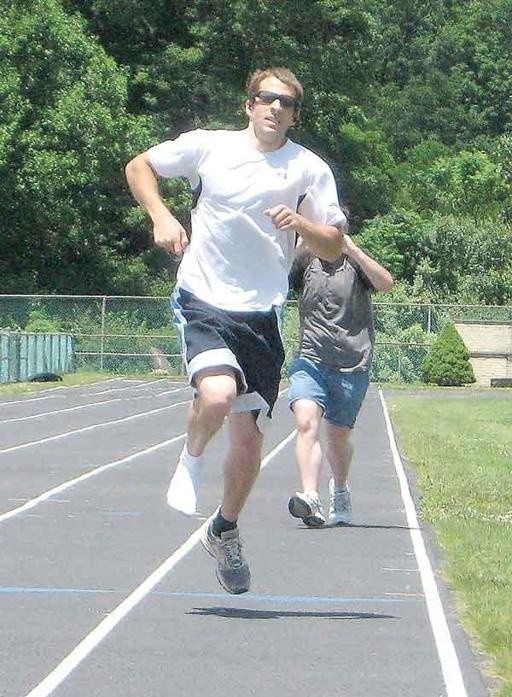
[253,91,296,108]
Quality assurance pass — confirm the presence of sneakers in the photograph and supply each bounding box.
[200,519,252,595]
[328,479,351,525]
[288,492,327,526]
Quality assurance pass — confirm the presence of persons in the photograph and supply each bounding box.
[124,59,349,598]
[281,199,396,532]
